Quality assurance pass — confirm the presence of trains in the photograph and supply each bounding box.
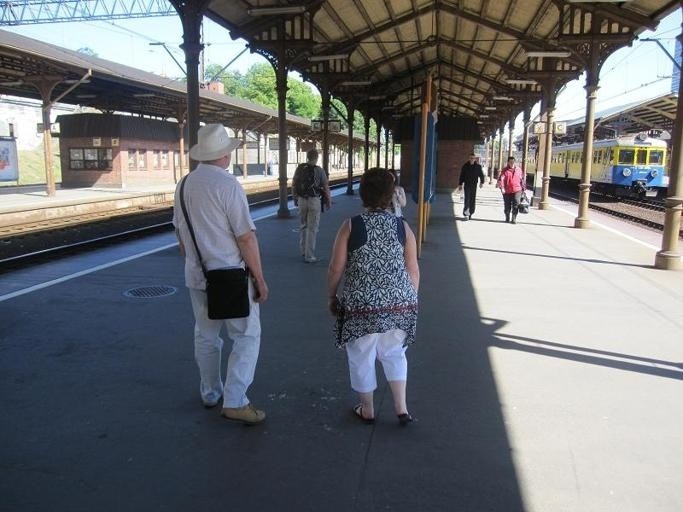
[474,132,670,201]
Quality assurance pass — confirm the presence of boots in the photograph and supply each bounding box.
[506,214,510,222]
[511,214,517,223]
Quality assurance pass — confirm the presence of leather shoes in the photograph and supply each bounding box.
[304,255,321,263]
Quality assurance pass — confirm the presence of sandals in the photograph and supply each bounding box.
[352,403,376,423]
[398,409,418,426]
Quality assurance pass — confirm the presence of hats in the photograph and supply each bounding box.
[189,123,241,161]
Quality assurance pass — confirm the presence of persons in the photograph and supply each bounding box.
[380,168,407,219]
[456,153,484,220]
[495,156,526,225]
[290,148,333,264]
[322,166,421,427]
[171,122,269,425]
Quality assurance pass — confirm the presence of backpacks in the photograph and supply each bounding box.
[293,163,324,200]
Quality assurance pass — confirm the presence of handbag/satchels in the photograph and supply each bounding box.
[205,267,250,320]
[519,191,530,214]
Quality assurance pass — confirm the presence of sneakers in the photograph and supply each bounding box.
[220,406,266,423]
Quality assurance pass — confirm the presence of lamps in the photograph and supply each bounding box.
[0,80,155,99]
[476,48,569,126]
[309,48,409,121]
